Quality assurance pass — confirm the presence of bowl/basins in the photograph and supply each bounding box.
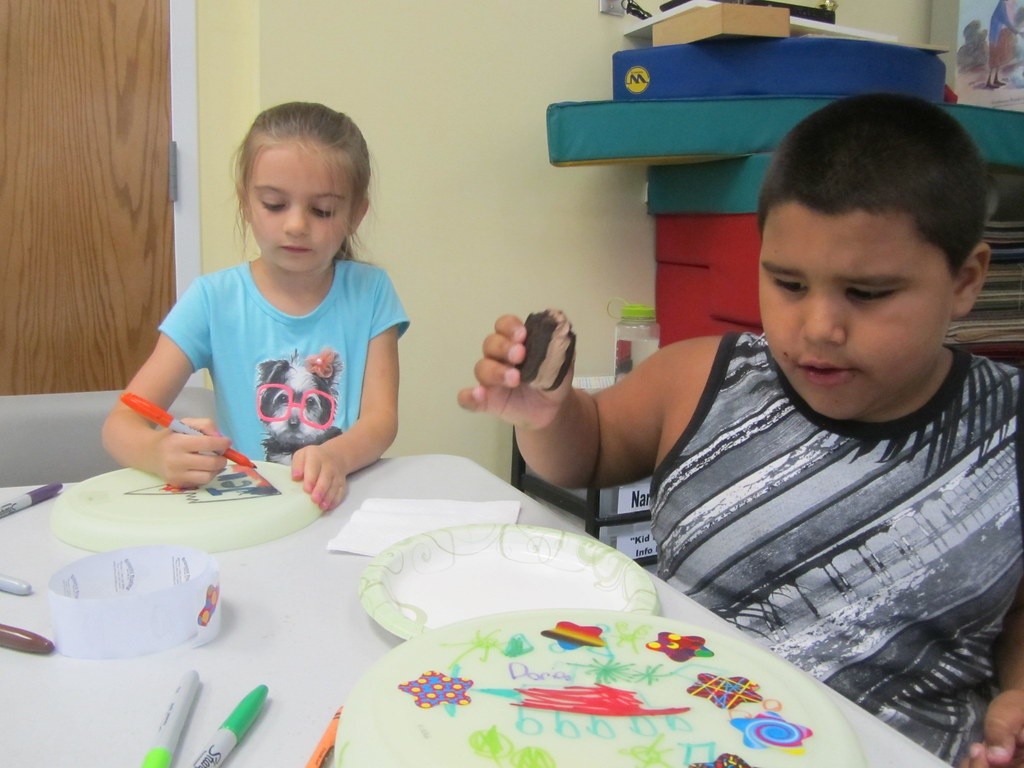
[44,546,221,658]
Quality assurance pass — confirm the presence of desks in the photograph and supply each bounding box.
[0,452,956,768]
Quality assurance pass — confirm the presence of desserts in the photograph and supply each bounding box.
[514,309,577,391]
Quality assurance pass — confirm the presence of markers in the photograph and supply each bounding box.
[0,574,32,597]
[139,670,199,768]
[0,482,64,519]
[304,706,341,768]
[190,684,268,768]
[0,624,55,653]
[118,392,258,473]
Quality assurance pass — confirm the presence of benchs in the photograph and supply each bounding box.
[0,386,222,489]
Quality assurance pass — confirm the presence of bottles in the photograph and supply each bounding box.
[613,304,661,383]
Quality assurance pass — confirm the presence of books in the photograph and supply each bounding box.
[945,220,1024,345]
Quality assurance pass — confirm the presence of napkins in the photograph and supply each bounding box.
[324,498,523,555]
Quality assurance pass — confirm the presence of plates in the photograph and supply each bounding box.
[360,522,659,648]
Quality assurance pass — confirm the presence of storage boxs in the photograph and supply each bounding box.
[535,0,1024,349]
[520,374,659,580]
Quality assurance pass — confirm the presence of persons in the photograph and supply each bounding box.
[103,102,408,513]
[458,94,1024,768]
